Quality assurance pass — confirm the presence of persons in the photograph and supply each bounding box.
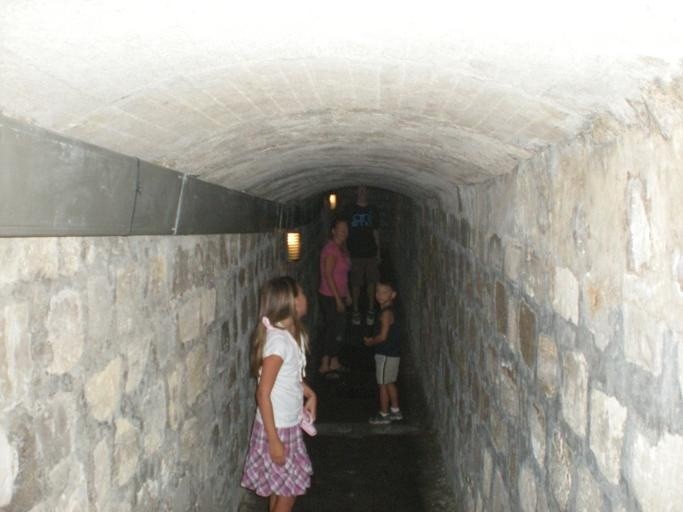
[340,183,382,327]
[240,275,319,512]
[316,216,356,381]
[363,274,404,425]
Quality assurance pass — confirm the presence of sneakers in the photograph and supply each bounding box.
[317,365,350,380]
[367,407,403,425]
[352,310,375,326]
